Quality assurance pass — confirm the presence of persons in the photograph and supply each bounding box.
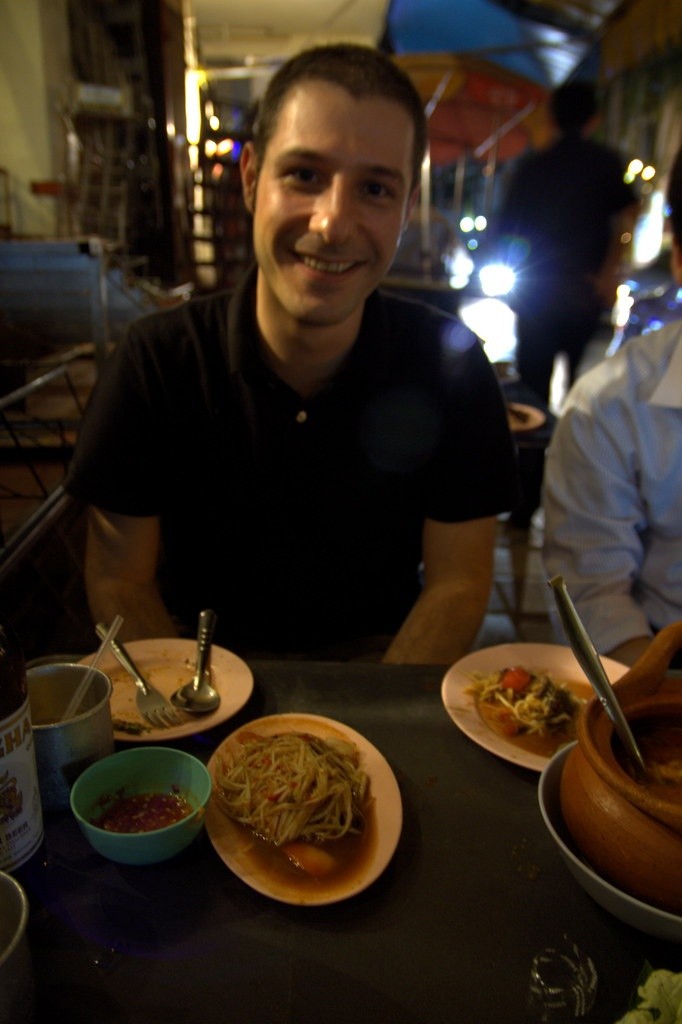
[542,141,681,676]
[498,77,642,529]
[62,45,521,665]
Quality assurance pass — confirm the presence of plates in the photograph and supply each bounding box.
[204,713,404,905]
[441,642,630,772]
[77,638,254,742]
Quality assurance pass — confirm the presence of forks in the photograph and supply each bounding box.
[96,622,182,728]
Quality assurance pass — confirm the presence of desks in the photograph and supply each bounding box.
[0,657,681,1024]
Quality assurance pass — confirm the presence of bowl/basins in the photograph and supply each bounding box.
[0,871,30,1024]
[70,746,212,866]
[537,739,682,937]
[26,663,115,815]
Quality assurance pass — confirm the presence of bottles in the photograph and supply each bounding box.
[0,622,48,886]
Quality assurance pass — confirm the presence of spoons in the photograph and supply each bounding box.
[170,609,219,713]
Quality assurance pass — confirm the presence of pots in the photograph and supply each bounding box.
[558,622,682,914]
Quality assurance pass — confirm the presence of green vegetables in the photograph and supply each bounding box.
[611,968,682,1024]
